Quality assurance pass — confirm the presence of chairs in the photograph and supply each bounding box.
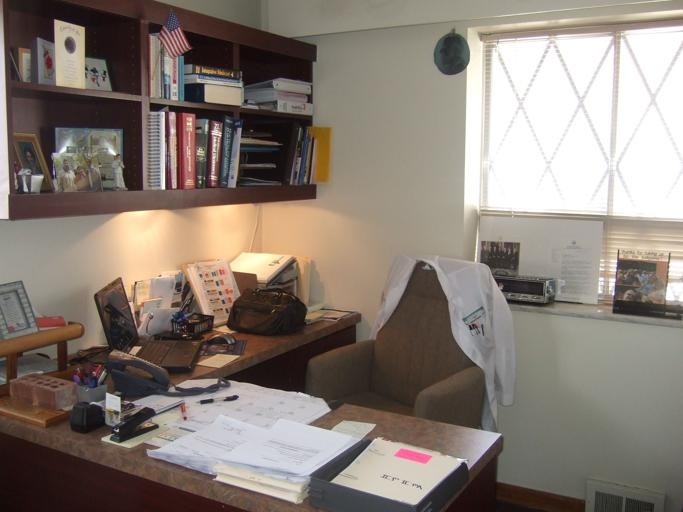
[305,253,514,430]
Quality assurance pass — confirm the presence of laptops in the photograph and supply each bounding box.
[94,277,203,371]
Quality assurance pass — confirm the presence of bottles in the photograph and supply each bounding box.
[136,311,153,336]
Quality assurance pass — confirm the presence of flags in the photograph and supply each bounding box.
[158,12,191,58]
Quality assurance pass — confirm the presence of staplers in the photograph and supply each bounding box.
[110,408,158,443]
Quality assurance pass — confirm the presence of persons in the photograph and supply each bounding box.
[616,268,665,306]
[480,244,519,269]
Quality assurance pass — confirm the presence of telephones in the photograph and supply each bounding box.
[108,349,169,394]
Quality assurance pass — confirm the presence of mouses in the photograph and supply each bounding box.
[207,332,236,345]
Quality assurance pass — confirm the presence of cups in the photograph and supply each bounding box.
[22,172,45,193]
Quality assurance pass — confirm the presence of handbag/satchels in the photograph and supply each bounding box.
[226,287,307,336]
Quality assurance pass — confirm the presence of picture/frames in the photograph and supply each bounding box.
[11,132,53,192]
[1,280,40,341]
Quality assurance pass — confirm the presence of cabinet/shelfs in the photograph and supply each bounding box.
[1,1,317,220]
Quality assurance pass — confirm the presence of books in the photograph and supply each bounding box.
[228,250,350,325]
[147,33,317,189]
[146,414,360,506]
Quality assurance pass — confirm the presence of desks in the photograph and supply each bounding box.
[1,307,503,511]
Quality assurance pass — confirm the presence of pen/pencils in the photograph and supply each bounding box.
[180,403,187,420]
[200,395,239,404]
[73,364,107,388]
